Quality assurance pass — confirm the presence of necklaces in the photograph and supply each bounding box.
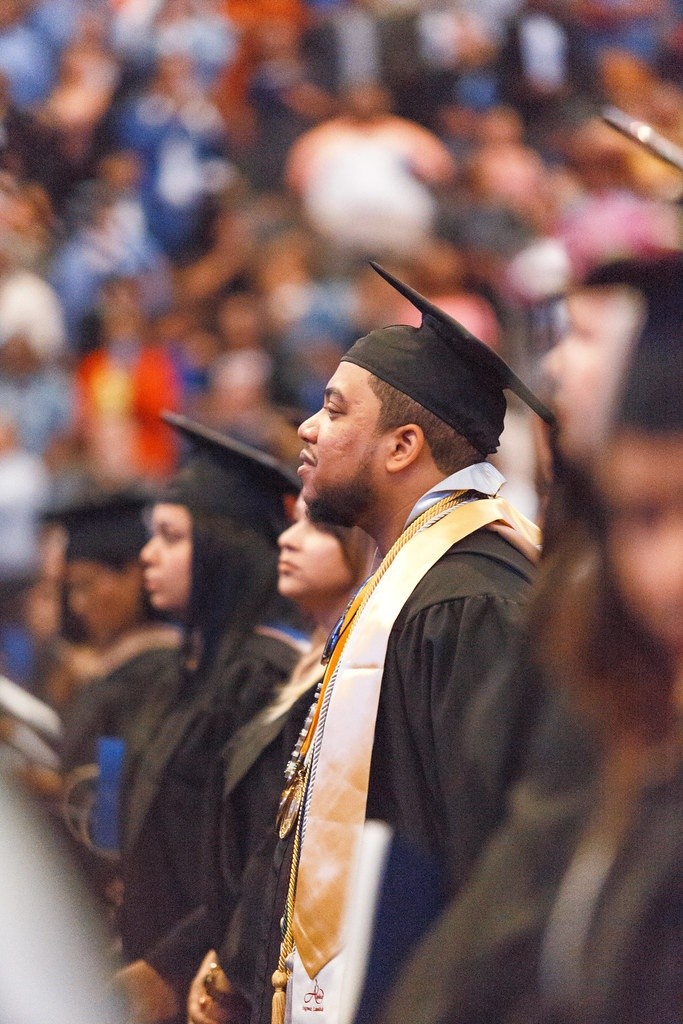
[320,572,377,665]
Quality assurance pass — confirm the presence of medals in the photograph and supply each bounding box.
[277,768,305,841]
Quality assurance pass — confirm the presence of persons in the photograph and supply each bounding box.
[0,0,683,1023]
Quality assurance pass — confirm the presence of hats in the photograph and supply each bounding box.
[341,261,557,457]
[147,408,301,544]
[587,255,683,424]
[42,496,154,567]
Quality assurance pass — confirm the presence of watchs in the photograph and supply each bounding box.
[202,962,251,1017]
